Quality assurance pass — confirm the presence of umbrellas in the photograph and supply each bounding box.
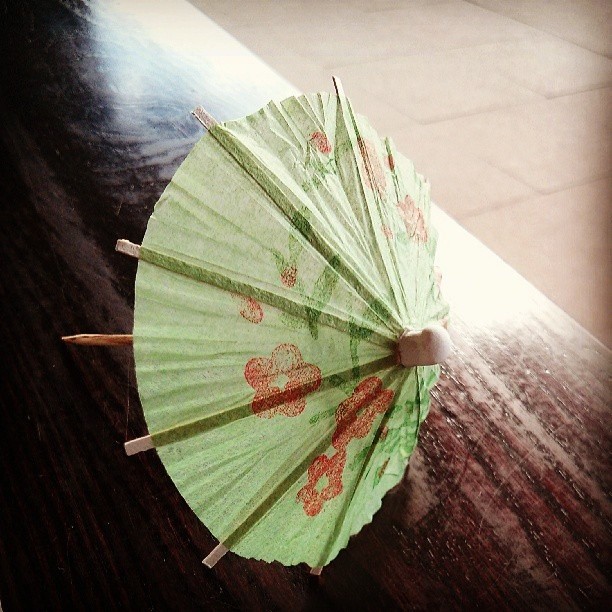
[61,73,452,569]
